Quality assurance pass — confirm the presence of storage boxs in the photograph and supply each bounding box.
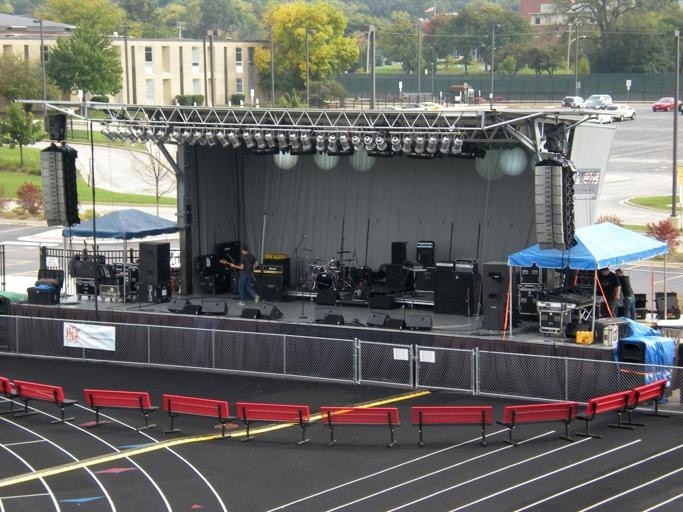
[27,269,64,304]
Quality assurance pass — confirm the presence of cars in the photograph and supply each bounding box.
[561,94,636,124]
[652,97,683,111]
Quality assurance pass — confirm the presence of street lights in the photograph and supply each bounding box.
[566,23,587,96]
[264,24,275,107]
[123,23,134,104]
[664,30,680,216]
[416,17,423,96]
[33,19,46,115]
[490,23,501,102]
[305,29,315,107]
[360,24,376,109]
[202,30,213,106]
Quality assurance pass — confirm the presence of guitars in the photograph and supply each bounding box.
[220,259,254,273]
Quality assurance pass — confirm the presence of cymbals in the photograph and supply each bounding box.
[343,259,354,261]
[337,251,351,254]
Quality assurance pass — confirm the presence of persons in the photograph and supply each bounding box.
[615,269,636,321]
[596,268,621,317]
[229,243,260,306]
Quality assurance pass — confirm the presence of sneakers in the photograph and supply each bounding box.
[236,295,259,306]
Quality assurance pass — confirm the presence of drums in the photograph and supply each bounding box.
[329,258,339,270]
[316,270,339,291]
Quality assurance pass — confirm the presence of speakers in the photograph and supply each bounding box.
[416,241,435,266]
[480,262,509,331]
[391,241,407,265]
[136,240,170,303]
[533,156,578,250]
[40,141,80,227]
[168,290,434,330]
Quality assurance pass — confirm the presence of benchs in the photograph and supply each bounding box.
[574,390,636,440]
[496,402,580,446]
[319,406,404,446]
[411,406,494,446]
[236,403,318,446]
[13,379,78,425]
[1,376,25,415]
[80,388,161,432]
[621,378,668,427]
[163,394,236,440]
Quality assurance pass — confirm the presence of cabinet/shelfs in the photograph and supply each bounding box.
[538,304,594,339]
